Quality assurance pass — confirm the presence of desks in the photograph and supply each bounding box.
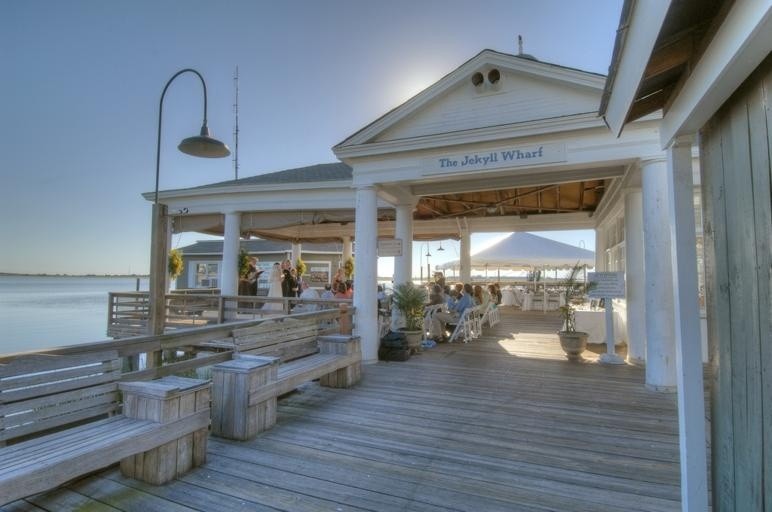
[569,303,622,346]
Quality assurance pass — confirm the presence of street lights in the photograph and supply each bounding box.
[147,67,233,369]
[420,238,446,286]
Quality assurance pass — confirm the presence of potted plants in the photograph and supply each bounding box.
[390,281,432,350]
[555,263,604,363]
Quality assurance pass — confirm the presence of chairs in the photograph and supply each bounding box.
[447,295,501,345]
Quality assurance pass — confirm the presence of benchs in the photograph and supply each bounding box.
[2,375,210,510]
[210,334,365,449]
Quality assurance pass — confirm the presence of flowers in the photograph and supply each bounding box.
[168,249,184,278]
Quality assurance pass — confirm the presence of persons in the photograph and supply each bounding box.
[421,278,502,343]
[246,257,257,296]
[269,262,354,313]
[377,286,385,299]
[527,270,541,281]
